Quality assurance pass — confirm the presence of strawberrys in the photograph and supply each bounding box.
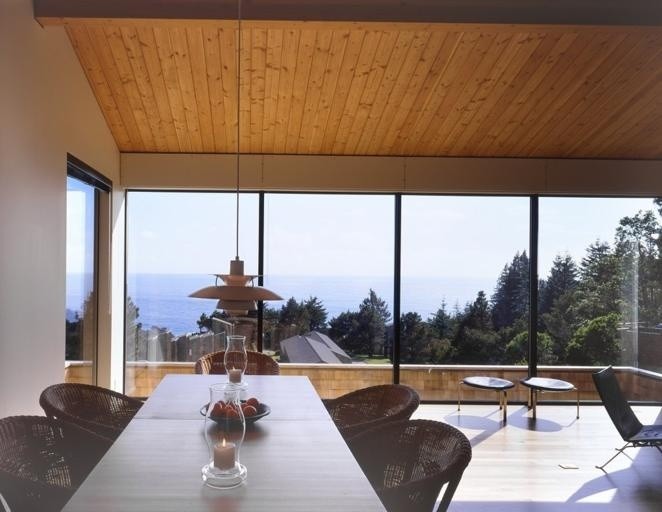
[206,397,259,417]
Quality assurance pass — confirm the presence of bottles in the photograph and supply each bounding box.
[223,335,247,391]
[201,382,247,491]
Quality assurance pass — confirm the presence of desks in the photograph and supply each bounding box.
[61,373,388,512]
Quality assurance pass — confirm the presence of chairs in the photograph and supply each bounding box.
[40,383,144,440]
[194,350,281,375]
[320,384,419,437]
[593,365,662,470]
[0,415,113,512]
[344,419,470,512]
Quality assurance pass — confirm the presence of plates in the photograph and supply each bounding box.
[199,400,271,426]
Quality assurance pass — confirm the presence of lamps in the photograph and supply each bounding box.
[187,2,284,318]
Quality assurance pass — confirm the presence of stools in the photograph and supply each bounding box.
[520,376,579,421]
[457,376,515,426]
